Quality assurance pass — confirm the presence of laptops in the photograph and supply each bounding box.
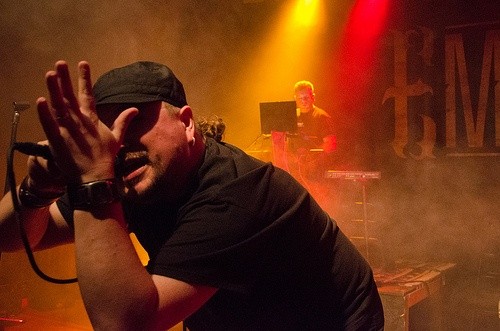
[260,101,298,134]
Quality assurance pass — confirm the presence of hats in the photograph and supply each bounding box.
[92,61,186,109]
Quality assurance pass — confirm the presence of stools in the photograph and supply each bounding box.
[379,262,456,331]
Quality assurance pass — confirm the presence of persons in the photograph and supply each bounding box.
[287,80,337,159]
[0,60,385,331]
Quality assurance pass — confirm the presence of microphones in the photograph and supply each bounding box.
[13,141,53,160]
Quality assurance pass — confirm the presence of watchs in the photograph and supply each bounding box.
[67,178,122,209]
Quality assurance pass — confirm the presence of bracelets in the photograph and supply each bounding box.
[19,175,64,208]
